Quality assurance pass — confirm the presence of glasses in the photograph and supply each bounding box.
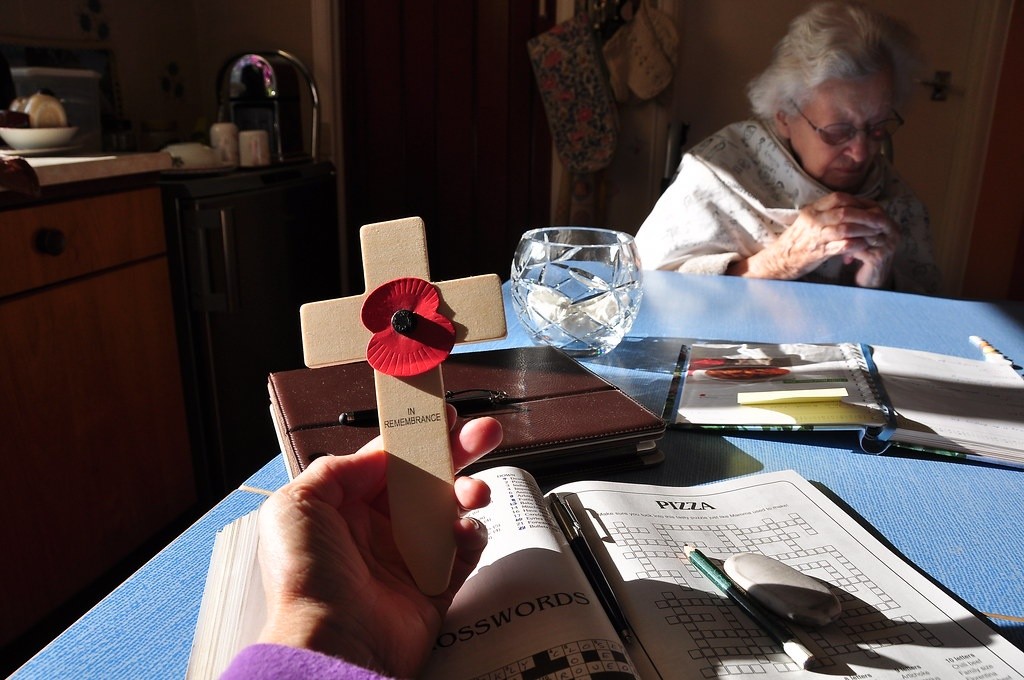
[790,99,905,146]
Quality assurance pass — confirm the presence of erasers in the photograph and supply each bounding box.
[724,552,841,626]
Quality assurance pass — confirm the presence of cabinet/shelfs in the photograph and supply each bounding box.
[0,187,199,649]
[157,155,342,502]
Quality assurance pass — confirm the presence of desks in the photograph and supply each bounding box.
[5,261,1024,680]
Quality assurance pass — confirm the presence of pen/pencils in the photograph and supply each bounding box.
[683,542,815,668]
[548,493,632,649]
[339,388,510,424]
[970,336,1014,367]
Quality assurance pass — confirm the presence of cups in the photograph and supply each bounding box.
[510,226,645,359]
[239,130,270,166]
[210,122,240,162]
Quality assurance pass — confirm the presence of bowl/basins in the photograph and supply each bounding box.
[0,127,81,150]
[159,143,225,168]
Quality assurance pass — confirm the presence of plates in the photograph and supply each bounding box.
[0,144,81,154]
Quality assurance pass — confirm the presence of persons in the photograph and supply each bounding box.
[219,403,502,680]
[635,0,945,295]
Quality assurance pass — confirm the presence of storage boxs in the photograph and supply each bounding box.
[9,67,103,153]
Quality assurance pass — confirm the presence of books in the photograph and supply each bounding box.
[187,345,1024,680]
